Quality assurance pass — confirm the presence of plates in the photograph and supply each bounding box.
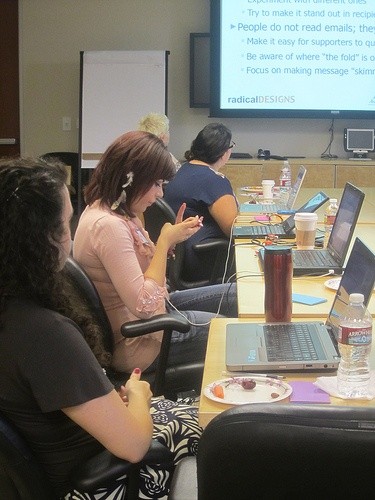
[203,375,292,404]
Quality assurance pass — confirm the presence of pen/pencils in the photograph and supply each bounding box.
[223,372,285,380]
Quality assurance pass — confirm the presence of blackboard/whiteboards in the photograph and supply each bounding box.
[79,50,170,168]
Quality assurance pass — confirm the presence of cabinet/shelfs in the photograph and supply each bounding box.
[219,158,375,224]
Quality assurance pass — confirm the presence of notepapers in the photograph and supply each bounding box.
[292,382,332,404]
[292,293,327,307]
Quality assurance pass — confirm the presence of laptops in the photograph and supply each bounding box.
[225,165,375,371]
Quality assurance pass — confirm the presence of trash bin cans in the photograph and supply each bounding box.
[43,151,90,213]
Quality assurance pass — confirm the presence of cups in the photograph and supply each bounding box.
[262,179,275,199]
[293,213,319,249]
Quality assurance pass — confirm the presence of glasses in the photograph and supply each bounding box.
[228,140,236,149]
[251,240,266,247]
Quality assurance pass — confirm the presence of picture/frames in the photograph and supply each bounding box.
[189,32,211,108]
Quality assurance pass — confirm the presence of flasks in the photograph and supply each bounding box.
[264,245,292,323]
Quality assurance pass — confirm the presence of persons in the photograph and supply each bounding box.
[0,159,203,500]
[139,113,182,185]
[72,131,237,380]
[161,121,239,283]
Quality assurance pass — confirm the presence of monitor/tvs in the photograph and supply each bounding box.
[344,128,375,152]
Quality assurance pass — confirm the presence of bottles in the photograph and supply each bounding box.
[323,198,338,249]
[336,293,372,398]
[279,160,291,193]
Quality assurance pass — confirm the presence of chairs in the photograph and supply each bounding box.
[40,149,91,216]
[196,403,375,500]
[0,198,235,500]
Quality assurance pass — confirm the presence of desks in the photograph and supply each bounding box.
[197,188,375,427]
